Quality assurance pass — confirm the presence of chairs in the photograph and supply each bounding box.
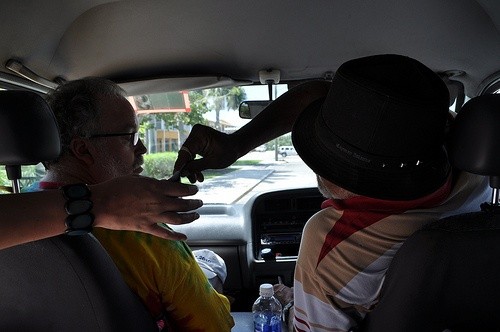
[357,90,500,332]
[0,89,160,332]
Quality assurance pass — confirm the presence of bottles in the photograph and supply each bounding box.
[252,284,283,332]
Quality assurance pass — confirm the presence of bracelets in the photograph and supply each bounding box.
[59,182,95,237]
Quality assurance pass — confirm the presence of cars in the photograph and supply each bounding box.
[255,144,267,152]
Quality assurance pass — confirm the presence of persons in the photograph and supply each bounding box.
[38,75,235,332]
[0,173,203,252]
[174,53,492,332]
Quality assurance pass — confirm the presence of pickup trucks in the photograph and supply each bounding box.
[278,145,299,157]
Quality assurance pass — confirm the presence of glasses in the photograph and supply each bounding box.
[85,132,139,146]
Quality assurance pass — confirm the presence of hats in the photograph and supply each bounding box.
[290,54,454,201]
